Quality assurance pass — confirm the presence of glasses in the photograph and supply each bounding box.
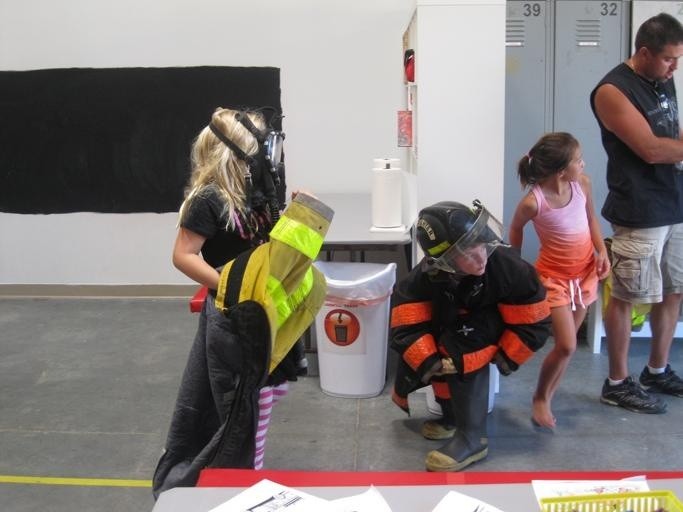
[265,129,286,169]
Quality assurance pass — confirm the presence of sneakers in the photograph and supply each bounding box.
[600,375,670,414]
[639,364,682,397]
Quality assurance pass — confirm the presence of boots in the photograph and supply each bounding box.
[425,373,489,472]
[416,393,453,439]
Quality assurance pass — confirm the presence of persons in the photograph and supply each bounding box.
[390,198,551,472]
[172,107,309,469]
[589,12,683,414]
[509,132,611,428]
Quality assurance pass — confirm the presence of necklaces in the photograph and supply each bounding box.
[631,56,640,76]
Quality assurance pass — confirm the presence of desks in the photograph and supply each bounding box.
[300,192,412,355]
[147,476,681,512]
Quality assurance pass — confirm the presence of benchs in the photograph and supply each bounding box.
[583,267,682,352]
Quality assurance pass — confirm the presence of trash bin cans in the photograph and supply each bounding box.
[312,261,397,399]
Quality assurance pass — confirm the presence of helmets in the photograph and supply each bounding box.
[406,198,500,275]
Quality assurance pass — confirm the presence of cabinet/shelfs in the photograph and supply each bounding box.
[505,1,555,268]
[552,2,632,350]
[631,1,682,138]
